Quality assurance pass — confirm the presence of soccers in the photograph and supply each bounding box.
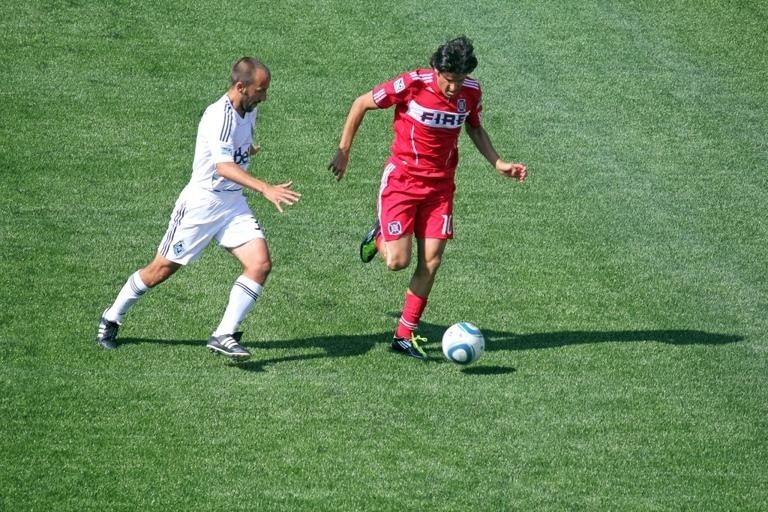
[442,322,485,365]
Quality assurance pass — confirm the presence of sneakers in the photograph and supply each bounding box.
[360,219,381,263]
[95,307,123,350]
[206,331,252,362]
[387,331,429,362]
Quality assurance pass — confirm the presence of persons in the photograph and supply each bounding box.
[96,59,302,361]
[327,35,528,359]
